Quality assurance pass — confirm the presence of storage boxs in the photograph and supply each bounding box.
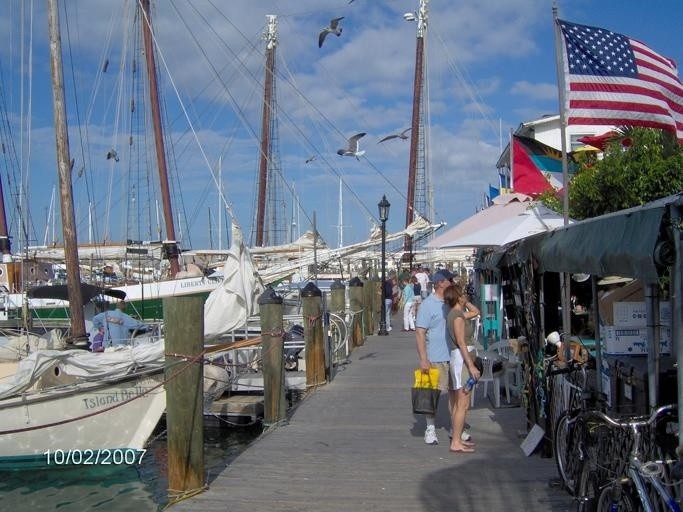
[598,276,671,358]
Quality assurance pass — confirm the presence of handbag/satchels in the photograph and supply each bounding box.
[410,368,442,415]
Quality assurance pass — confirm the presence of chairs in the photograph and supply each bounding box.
[469,338,527,409]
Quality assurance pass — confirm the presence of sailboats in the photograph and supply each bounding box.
[0,0,444,470]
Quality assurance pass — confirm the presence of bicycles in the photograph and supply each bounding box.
[554,363,683,512]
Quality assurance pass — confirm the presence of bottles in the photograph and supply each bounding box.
[463,376,477,393]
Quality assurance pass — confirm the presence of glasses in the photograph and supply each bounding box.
[444,278,453,282]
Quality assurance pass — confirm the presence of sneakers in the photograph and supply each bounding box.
[424,429,438,445]
[448,429,472,441]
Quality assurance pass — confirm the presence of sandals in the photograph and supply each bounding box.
[450,441,476,453]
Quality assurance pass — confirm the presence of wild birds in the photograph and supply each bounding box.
[336,132,367,162]
[377,128,413,144]
[319,17,345,49]
[306,156,317,164]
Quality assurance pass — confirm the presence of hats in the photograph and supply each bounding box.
[431,269,459,283]
[596,275,634,286]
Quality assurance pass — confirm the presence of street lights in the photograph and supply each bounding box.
[378,193,392,337]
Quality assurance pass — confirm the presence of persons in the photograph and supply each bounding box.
[93,301,152,349]
[385,266,482,453]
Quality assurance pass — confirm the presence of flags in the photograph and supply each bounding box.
[512,134,582,195]
[556,17,683,145]
[486,173,510,208]
